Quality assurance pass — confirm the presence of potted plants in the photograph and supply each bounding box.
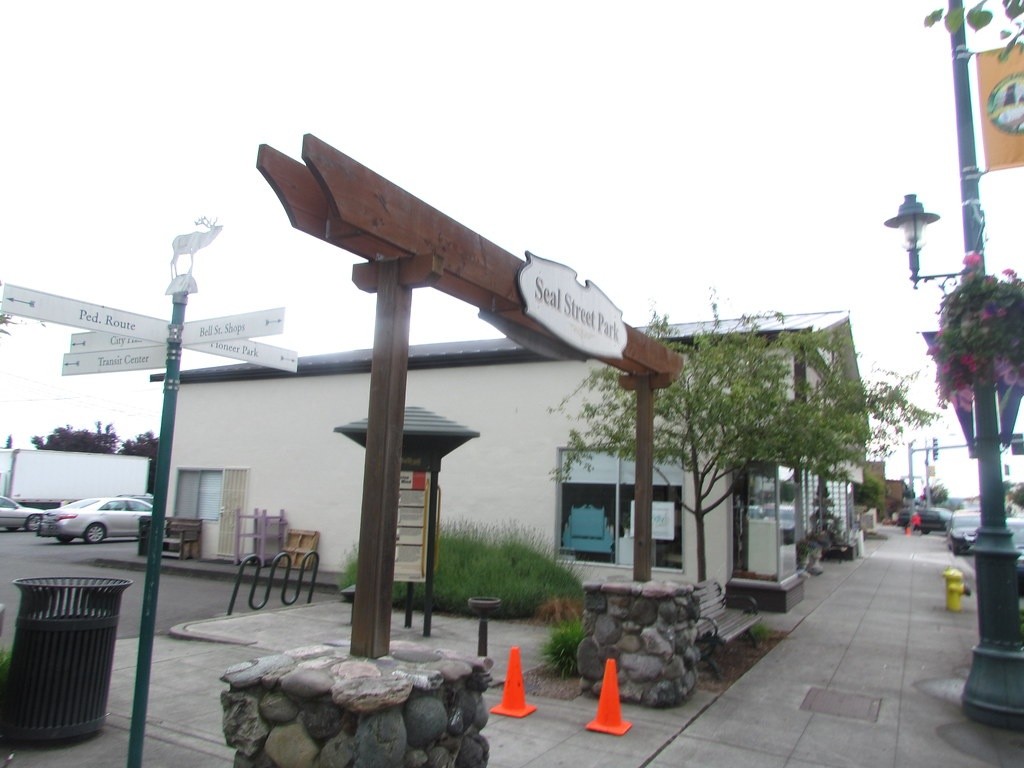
[798,544,812,570]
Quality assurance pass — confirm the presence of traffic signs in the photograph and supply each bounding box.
[0,283,299,374]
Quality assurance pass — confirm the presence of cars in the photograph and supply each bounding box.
[0,494,154,544]
[896,508,1023,576]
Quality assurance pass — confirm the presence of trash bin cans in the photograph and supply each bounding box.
[1,576,134,740]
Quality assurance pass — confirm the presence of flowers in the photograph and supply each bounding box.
[927,250,1024,412]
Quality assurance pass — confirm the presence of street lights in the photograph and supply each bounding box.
[884,1,1024,730]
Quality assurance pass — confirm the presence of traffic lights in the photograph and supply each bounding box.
[932,438,940,461]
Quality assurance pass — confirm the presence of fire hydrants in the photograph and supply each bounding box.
[942,569,973,612]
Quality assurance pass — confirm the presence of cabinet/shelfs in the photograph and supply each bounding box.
[233,507,289,567]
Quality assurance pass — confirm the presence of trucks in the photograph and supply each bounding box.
[0,448,154,509]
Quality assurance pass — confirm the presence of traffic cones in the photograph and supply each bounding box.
[491,647,539,723]
[586,659,634,737]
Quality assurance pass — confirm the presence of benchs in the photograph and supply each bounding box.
[161,516,203,560]
[691,578,765,682]
